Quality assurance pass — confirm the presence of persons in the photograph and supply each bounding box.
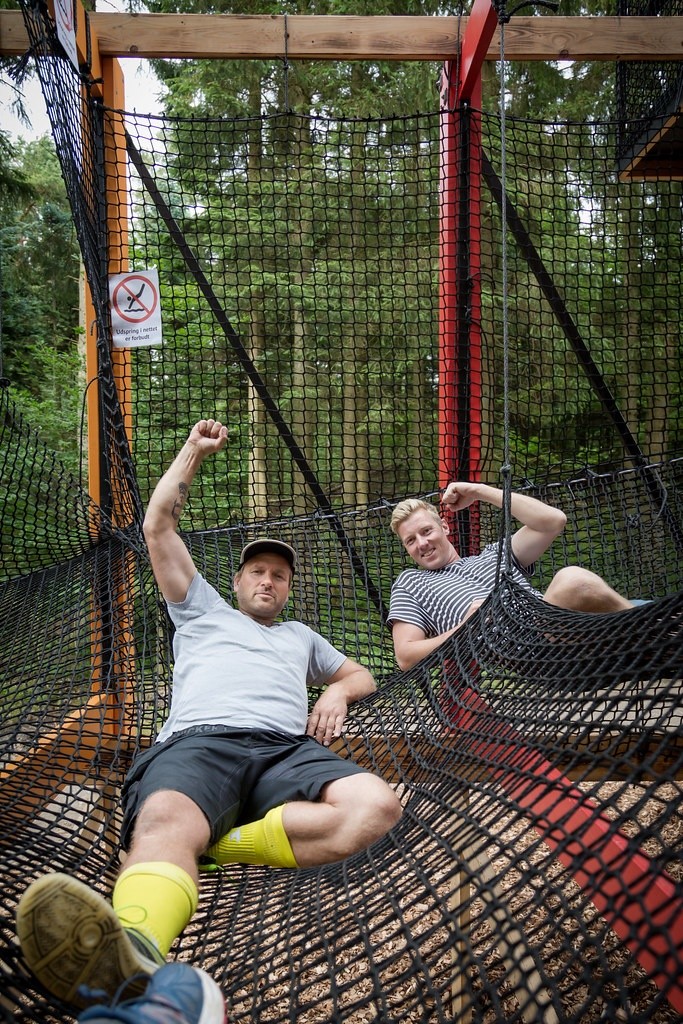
[76,962,228,1024]
[387,482,683,671]
[17,419,403,1013]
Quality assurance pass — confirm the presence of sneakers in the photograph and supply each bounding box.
[15,870,163,1008]
[77,961,227,1024]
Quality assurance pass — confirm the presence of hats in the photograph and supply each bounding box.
[237,538,298,582]
